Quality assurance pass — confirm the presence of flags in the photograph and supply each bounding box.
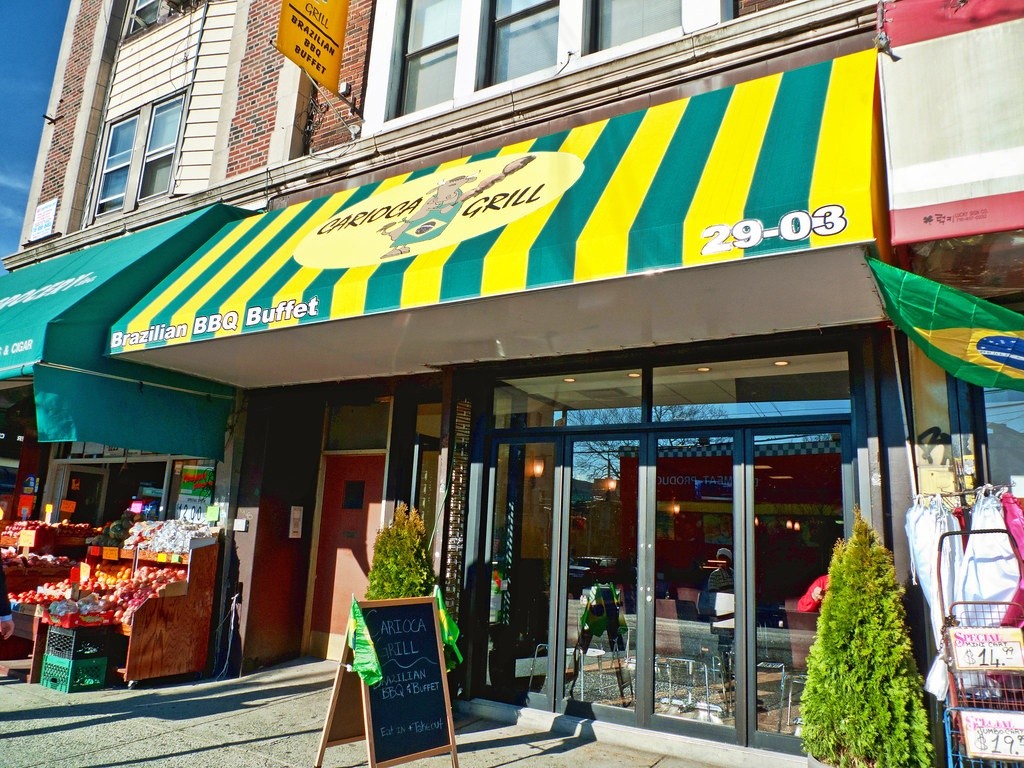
[865,252,1024,392]
[433,587,465,671]
[580,579,630,637]
[349,595,383,687]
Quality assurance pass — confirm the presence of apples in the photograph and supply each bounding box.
[8,560,188,625]
[1,518,112,568]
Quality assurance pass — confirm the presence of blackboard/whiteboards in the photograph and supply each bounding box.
[323,595,456,768]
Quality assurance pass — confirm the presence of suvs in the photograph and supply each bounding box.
[569,557,620,598]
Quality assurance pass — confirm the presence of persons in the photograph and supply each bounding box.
[709,546,737,682]
[0,549,16,641]
[797,573,832,614]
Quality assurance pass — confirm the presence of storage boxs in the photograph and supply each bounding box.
[40,653,109,692]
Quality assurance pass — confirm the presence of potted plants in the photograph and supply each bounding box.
[795,506,939,768]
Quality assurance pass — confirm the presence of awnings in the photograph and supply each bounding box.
[109,45,901,394]
[0,200,238,463]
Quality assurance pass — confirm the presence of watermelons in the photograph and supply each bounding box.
[95,519,130,547]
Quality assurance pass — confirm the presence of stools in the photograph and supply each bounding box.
[506,641,808,738]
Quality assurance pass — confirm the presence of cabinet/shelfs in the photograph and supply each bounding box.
[0,515,224,689]
